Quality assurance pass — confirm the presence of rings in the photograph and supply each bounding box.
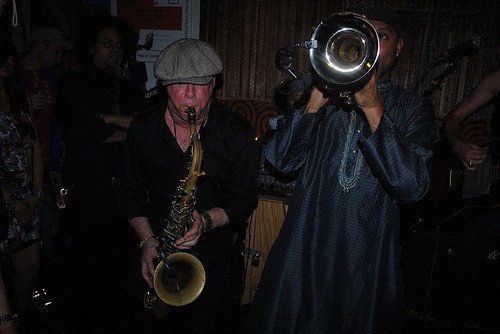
[469,160,472,167]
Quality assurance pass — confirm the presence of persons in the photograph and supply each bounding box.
[27,26,65,164]
[71,14,147,334]
[0,25,46,311]
[247,6,436,334]
[0,275,18,334]
[117,38,259,334]
[441,68,500,170]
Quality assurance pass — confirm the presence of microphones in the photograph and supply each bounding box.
[287,40,318,51]
[435,35,481,63]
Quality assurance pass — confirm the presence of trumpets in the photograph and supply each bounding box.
[306,10,381,105]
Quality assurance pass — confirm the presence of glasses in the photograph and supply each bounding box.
[95,40,121,49]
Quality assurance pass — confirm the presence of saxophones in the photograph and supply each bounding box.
[142,105,208,309]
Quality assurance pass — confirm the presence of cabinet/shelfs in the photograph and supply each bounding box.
[241,199,289,306]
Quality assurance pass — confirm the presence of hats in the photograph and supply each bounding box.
[154,37,223,86]
[365,7,404,38]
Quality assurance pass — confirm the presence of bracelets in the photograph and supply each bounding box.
[6,194,17,209]
[31,190,42,199]
[201,212,212,231]
[0,311,18,325]
[140,235,155,249]
